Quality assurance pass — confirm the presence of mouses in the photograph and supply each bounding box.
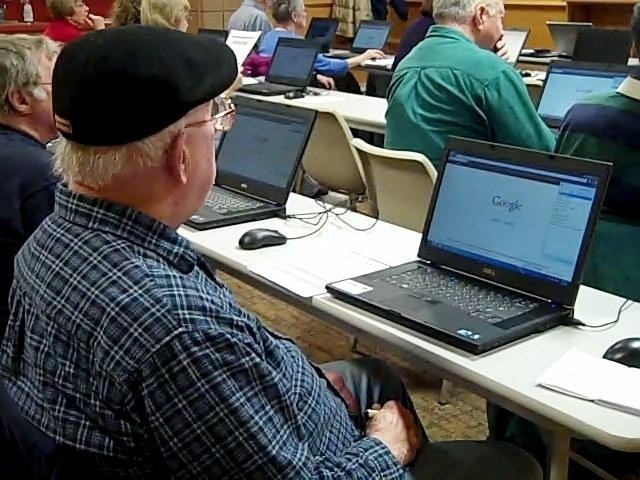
[285,90,304,99]
[603,335,640,369]
[238,227,287,250]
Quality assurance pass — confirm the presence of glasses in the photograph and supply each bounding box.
[184,96,237,133]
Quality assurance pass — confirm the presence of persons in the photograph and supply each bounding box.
[227,0,273,46]
[42,0,105,44]
[549,2,640,306]
[0,32,62,327]
[392,0,510,74]
[0,25,423,480]
[258,0,385,94]
[139,0,190,32]
[385,0,555,173]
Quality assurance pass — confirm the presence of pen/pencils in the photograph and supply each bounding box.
[363,408,380,418]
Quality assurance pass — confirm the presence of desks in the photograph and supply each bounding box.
[518,47,639,71]
[331,48,393,78]
[176,192,640,479]
[234,84,388,135]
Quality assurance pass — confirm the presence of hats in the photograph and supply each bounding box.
[51,23,238,147]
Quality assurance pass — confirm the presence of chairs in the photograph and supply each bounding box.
[347,138,454,406]
[299,107,367,195]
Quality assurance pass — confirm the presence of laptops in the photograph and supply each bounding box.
[351,19,392,53]
[197,28,228,43]
[304,16,340,53]
[502,28,531,67]
[234,36,321,96]
[536,66,629,130]
[326,136,614,356]
[545,20,593,56]
[181,92,320,232]
[571,28,633,65]
